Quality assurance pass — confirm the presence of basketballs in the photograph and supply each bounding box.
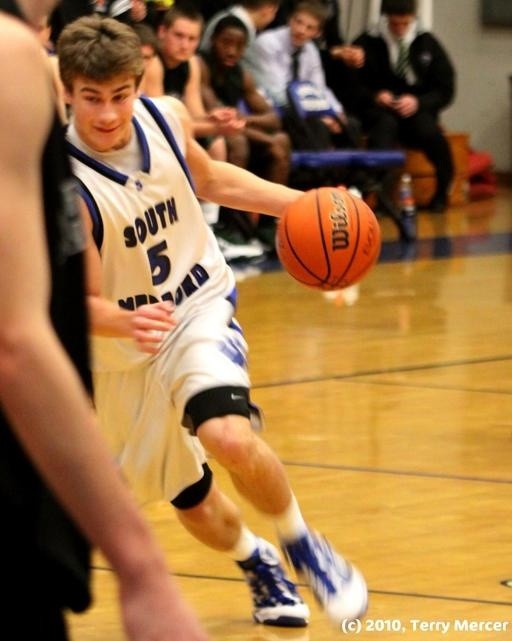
[274,185,382,290]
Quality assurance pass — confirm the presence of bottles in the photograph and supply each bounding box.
[394,174,419,244]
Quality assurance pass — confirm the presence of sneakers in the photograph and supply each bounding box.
[234,538,309,629]
[279,531,368,624]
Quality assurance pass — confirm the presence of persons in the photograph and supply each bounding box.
[3,0,208,640]
[54,18,373,626]
[2,0,457,260]
